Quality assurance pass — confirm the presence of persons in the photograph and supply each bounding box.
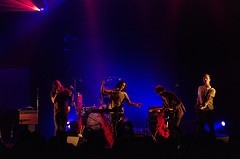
[196,74,216,140]
[50,79,72,137]
[99,79,111,108]
[153,85,186,152]
[100,80,143,148]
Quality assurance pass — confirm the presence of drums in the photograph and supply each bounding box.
[82,110,103,130]
